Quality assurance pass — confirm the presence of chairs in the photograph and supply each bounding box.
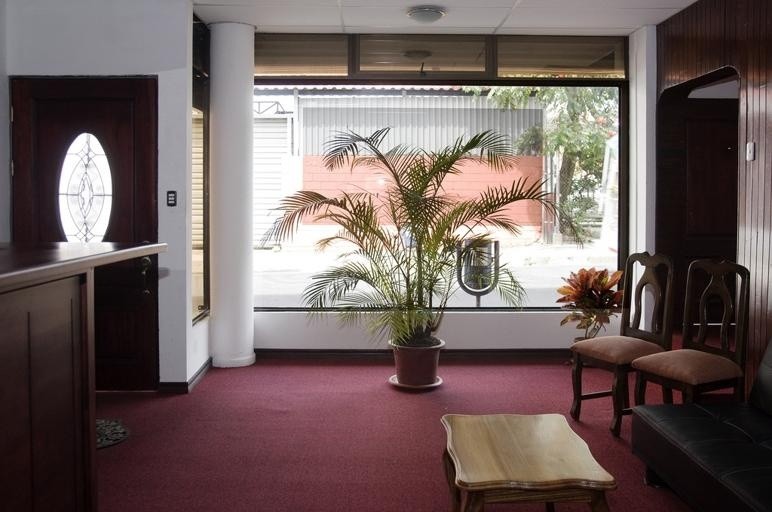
[568,250,673,437]
[630,252,751,412]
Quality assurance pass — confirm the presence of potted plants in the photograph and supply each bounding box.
[555,267,626,370]
[250,127,583,394]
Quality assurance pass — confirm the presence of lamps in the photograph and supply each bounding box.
[406,6,448,24]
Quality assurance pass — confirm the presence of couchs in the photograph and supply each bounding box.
[633,329,772,512]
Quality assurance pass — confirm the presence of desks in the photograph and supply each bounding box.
[0,242,170,512]
